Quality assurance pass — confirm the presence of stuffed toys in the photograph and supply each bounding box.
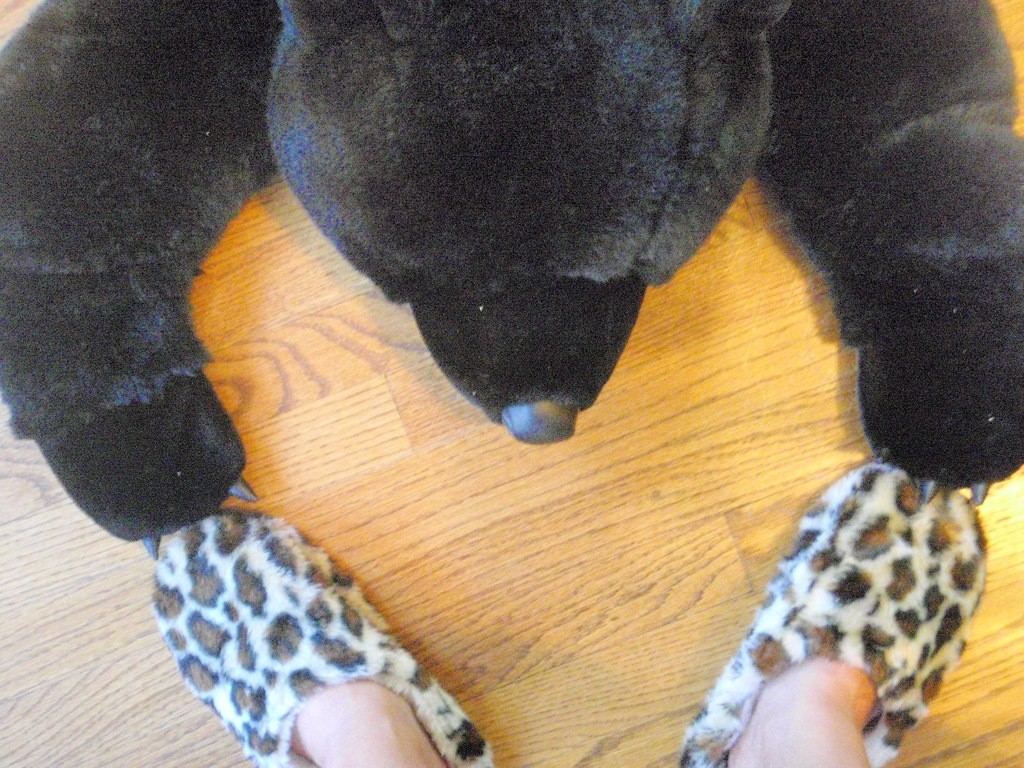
[0,0,1024,560]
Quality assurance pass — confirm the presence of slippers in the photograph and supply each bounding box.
[676,458,992,768]
[149,510,495,768]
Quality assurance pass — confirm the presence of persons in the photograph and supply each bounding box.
[152,460,987,768]
